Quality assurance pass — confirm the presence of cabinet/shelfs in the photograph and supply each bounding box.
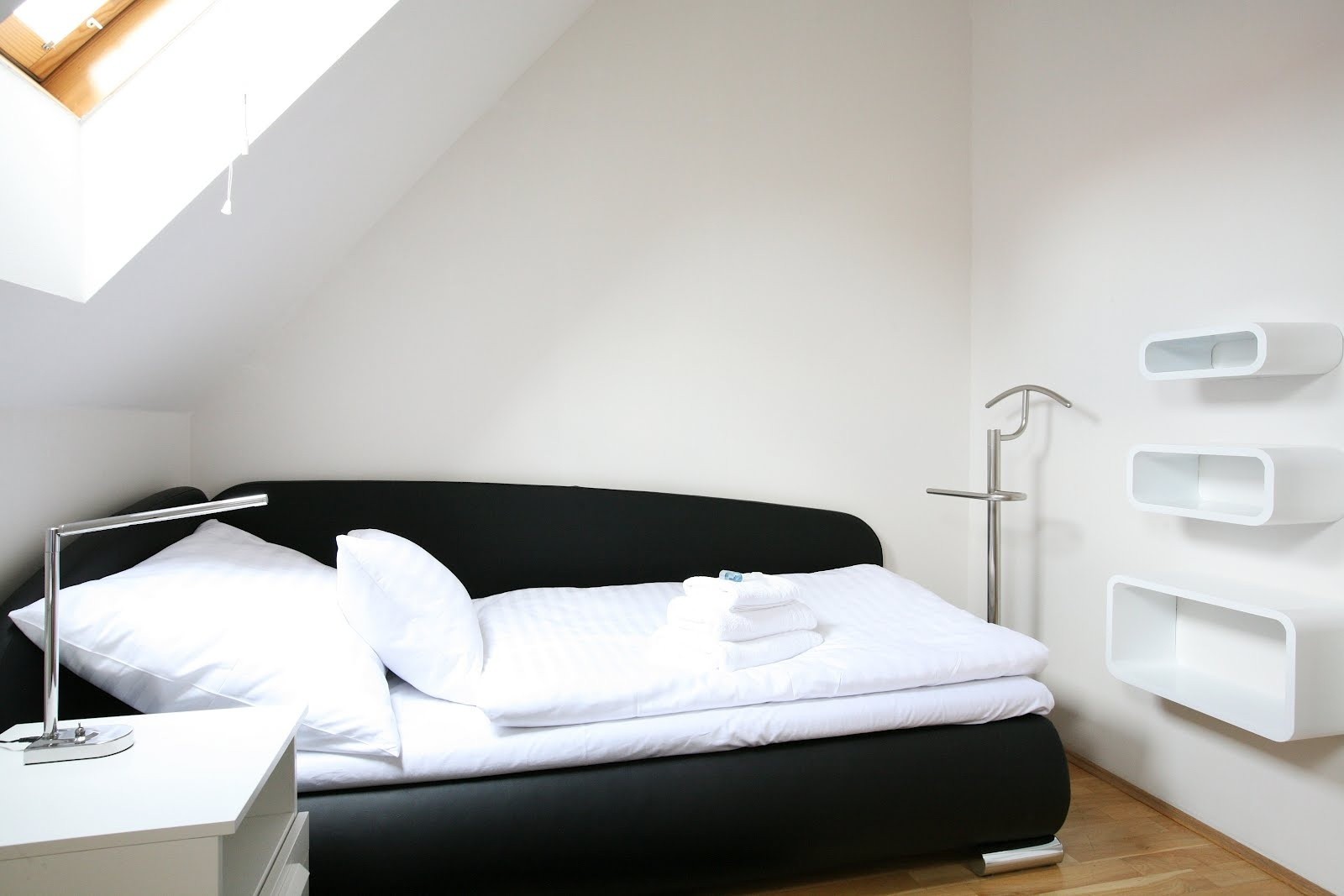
[1108,318,1344,743]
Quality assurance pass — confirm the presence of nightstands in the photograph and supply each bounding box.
[0,701,311,896]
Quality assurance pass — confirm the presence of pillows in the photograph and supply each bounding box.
[8,517,401,760]
[333,528,489,707]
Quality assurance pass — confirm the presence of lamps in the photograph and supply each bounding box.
[23,491,269,766]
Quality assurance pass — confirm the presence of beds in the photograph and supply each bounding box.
[0,479,1072,896]
